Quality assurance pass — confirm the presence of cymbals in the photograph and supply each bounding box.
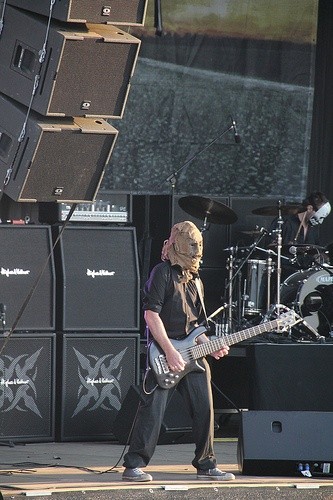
[179,195,238,224]
[253,205,299,217]
[271,242,325,252]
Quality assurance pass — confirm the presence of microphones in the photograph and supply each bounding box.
[260,227,271,235]
[231,116,241,143]
[289,246,307,256]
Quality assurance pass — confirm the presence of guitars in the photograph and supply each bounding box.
[148,317,289,389]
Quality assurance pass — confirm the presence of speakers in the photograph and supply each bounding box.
[135,194,299,337]
[236,409,333,478]
[0,223,141,446]
[0,0,148,205]
[114,389,220,445]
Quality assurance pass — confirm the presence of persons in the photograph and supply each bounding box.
[122,221,235,480]
[257,191,333,308]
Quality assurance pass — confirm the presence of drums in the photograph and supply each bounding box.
[281,268,333,337]
[233,257,276,316]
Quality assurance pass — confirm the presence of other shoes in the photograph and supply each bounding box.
[122,468,153,481]
[196,468,236,480]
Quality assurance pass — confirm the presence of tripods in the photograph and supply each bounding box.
[200,207,325,344]
